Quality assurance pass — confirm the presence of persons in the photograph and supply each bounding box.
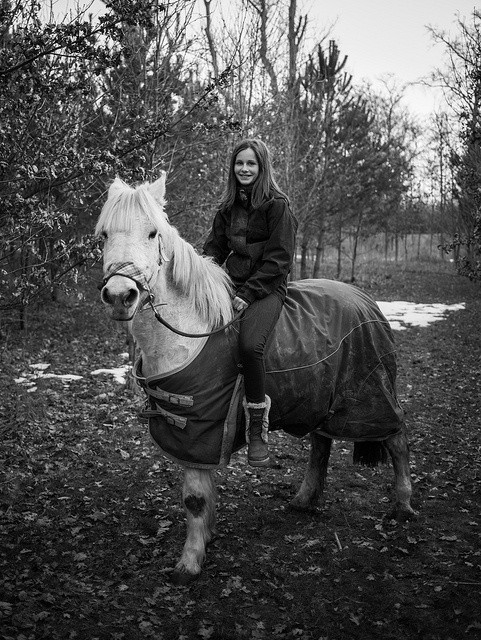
[202,138,299,467]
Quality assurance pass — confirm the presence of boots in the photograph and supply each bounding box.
[243,395,272,467]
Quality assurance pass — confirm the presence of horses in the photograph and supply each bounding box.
[94,168,417,580]
